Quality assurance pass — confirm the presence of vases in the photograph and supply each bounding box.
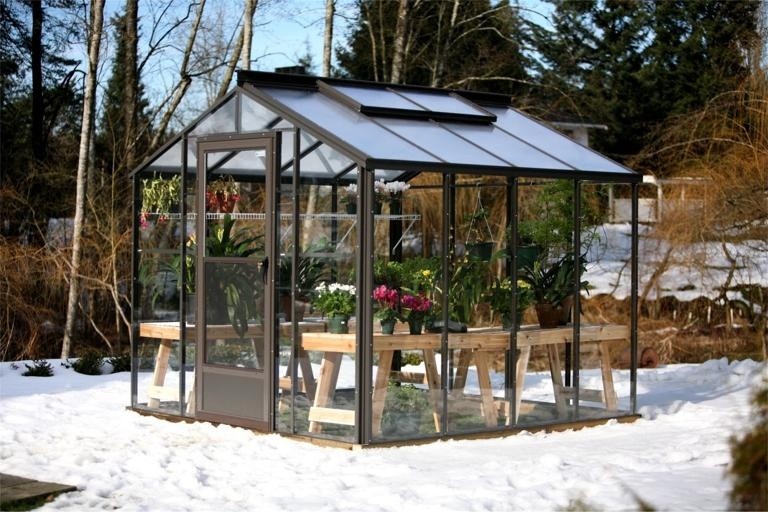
[380,319,397,335]
[327,313,350,333]
[409,320,423,335]
[502,313,523,331]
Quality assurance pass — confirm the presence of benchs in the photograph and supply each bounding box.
[139,318,432,416]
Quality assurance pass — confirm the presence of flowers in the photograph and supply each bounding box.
[373,285,399,320]
[206,173,241,211]
[312,281,356,317]
[136,171,182,231]
[482,276,535,315]
[401,289,429,320]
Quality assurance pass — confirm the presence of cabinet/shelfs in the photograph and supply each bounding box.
[300,322,629,439]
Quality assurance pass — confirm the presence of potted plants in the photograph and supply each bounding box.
[462,205,496,264]
[520,252,594,328]
[506,222,543,268]
[136,213,336,339]
